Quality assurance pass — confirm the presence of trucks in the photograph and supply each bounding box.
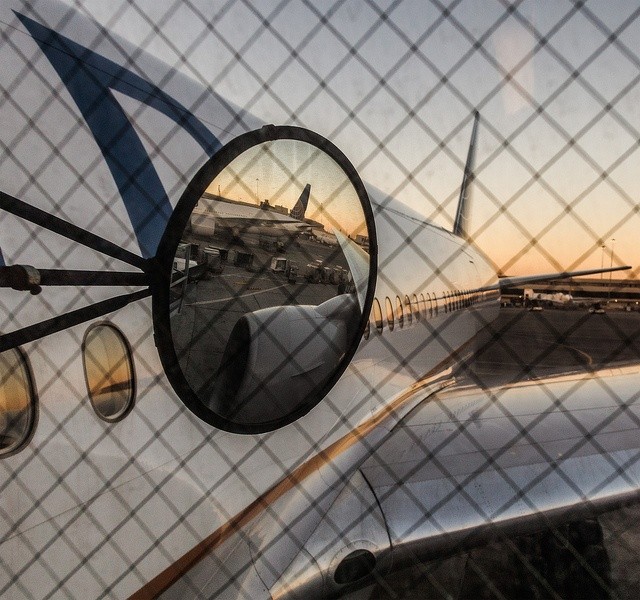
[271,257,297,275]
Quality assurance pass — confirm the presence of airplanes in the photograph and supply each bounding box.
[0,2,639,600]
[185,184,325,244]
